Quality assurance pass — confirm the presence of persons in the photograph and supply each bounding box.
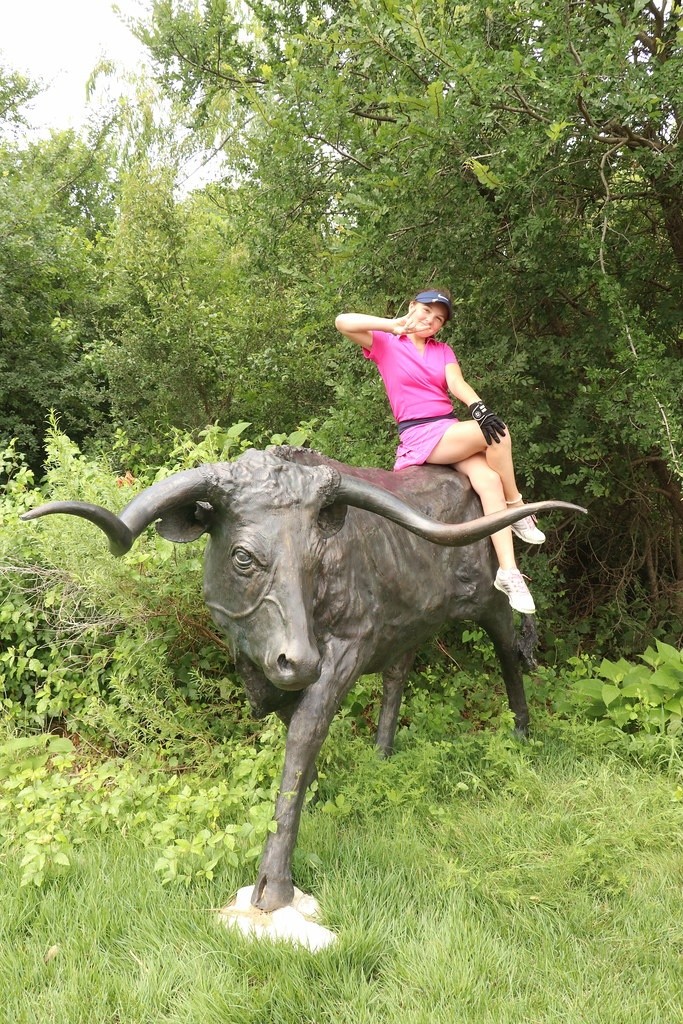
[335,287,547,614]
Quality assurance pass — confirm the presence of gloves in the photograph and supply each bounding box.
[470,401,506,445]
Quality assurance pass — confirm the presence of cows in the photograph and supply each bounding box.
[19,444,590,912]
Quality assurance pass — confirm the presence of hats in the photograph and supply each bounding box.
[415,291,452,321]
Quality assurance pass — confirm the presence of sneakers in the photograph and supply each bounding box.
[512,514,547,544]
[493,567,536,614]
